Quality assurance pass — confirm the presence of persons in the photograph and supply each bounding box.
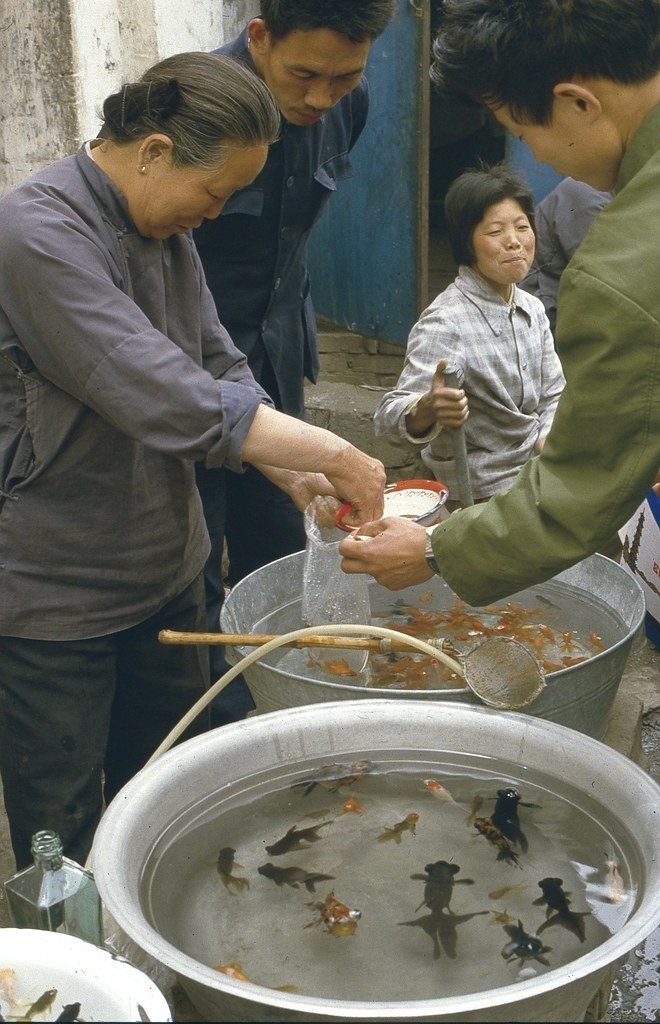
[340,0,660,608]
[192,0,399,730]
[0,48,386,927]
[517,177,614,340]
[374,161,567,512]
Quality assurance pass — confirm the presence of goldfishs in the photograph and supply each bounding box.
[204,757,627,969]
[8,988,84,1023]
[306,588,607,689]
[215,962,300,994]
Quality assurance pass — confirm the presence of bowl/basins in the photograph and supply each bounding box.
[218,548,647,742]
[90,699,660,1024]
[0,927,175,1024]
[333,478,450,543]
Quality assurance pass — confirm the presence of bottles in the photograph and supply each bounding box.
[3,829,104,950]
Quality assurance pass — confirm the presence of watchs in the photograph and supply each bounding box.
[426,524,439,574]
